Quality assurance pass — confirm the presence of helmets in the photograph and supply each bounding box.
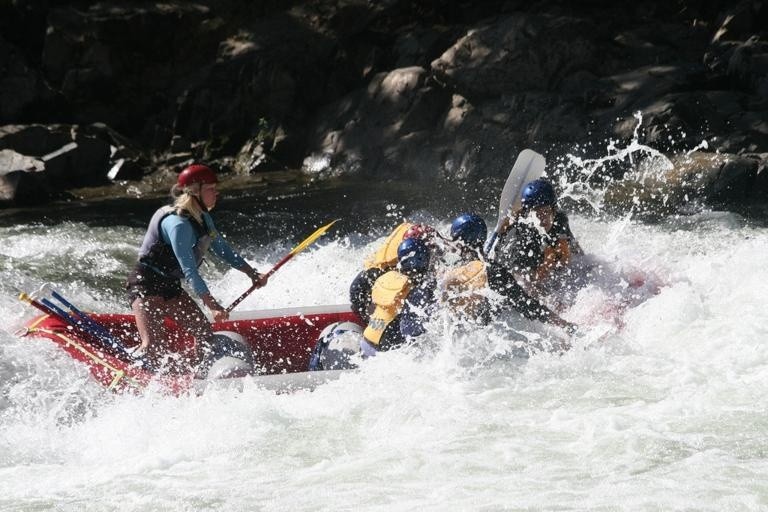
[521,179,556,208]
[450,214,487,246]
[178,165,217,188]
[397,238,430,270]
[403,224,438,240]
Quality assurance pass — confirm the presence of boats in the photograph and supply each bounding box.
[7,272,663,402]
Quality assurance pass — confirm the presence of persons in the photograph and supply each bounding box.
[130,165,266,359]
[155,329,255,376]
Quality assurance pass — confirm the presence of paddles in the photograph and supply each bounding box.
[485,149,546,257]
[224,217,341,312]
